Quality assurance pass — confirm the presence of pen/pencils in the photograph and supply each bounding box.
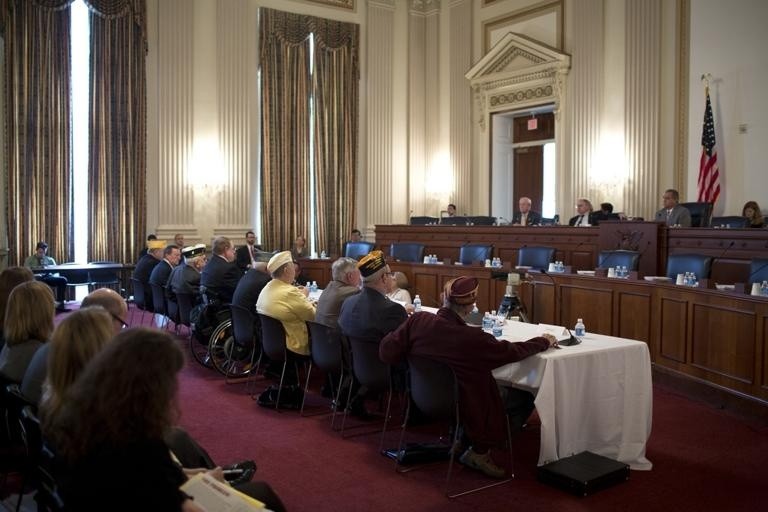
[222,469,243,473]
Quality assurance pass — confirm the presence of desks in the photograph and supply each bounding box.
[27,263,136,309]
[299,284,652,463]
[373,221,767,285]
[293,258,768,426]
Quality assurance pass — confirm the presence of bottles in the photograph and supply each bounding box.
[492,258,497,266]
[414,295,421,311]
[558,262,563,271]
[306,282,311,292]
[470,303,478,313]
[483,310,492,331]
[575,319,585,339]
[492,320,502,338]
[761,281,768,295]
[497,258,501,266]
[689,272,696,286]
[321,251,326,258]
[311,281,317,292]
[621,266,628,278]
[490,310,498,323]
[684,272,689,286]
[554,261,558,271]
[616,266,621,278]
[428,255,432,264]
[432,255,437,263]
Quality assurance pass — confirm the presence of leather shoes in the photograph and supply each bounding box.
[320,387,331,397]
[224,460,257,488]
[56,307,73,313]
[344,403,376,421]
[330,399,346,411]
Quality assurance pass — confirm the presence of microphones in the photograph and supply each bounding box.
[702,242,734,280]
[505,244,528,261]
[561,242,583,262]
[499,216,514,225]
[628,240,652,273]
[466,243,495,264]
[596,237,626,267]
[540,269,581,346]
[408,210,414,225]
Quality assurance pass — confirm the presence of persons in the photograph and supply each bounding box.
[132,247,162,312]
[291,236,310,260]
[170,244,208,325]
[379,275,558,477]
[338,250,434,426]
[293,262,313,286]
[601,203,613,215]
[38,307,256,486]
[42,329,288,512]
[23,242,71,313]
[569,198,627,228]
[742,201,768,227]
[256,250,338,399]
[316,258,381,421]
[448,204,457,217]
[174,233,187,249]
[1,267,34,351]
[655,190,691,228]
[237,232,262,268]
[200,236,242,305]
[512,197,542,226]
[385,271,413,306]
[229,253,274,363]
[21,287,128,405]
[138,234,156,259]
[0,280,56,383]
[149,245,182,316]
[342,230,362,257]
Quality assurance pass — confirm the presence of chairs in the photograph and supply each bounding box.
[250,311,309,410]
[465,216,497,227]
[596,249,642,271]
[665,253,715,285]
[337,329,401,452]
[45,435,112,508]
[518,246,556,273]
[166,287,200,337]
[297,321,348,430]
[62,262,88,305]
[225,302,274,399]
[393,354,516,500]
[15,402,45,512]
[711,216,745,229]
[147,280,166,331]
[4,383,37,511]
[680,202,712,227]
[126,277,151,326]
[346,242,372,257]
[441,217,465,227]
[88,261,124,299]
[459,246,494,266]
[747,256,768,286]
[410,216,439,224]
[538,215,560,224]
[390,242,424,263]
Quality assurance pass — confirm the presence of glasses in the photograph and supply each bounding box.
[386,272,395,278]
[112,314,128,329]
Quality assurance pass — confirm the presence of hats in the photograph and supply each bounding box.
[181,244,207,258]
[266,251,293,273]
[146,240,168,249]
[356,250,386,278]
[443,275,480,305]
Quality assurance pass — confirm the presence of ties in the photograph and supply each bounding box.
[667,211,670,220]
[576,215,584,225]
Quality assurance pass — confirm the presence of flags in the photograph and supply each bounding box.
[698,73,722,226]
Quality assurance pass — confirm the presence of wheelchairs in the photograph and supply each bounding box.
[189,286,263,378]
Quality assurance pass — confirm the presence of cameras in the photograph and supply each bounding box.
[490,270,534,285]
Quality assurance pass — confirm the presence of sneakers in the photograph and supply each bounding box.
[448,440,466,459]
[458,446,505,479]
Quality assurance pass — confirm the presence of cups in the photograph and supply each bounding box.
[549,263,554,272]
[750,283,762,296]
[676,274,683,285]
[608,269,614,278]
[484,260,491,266]
